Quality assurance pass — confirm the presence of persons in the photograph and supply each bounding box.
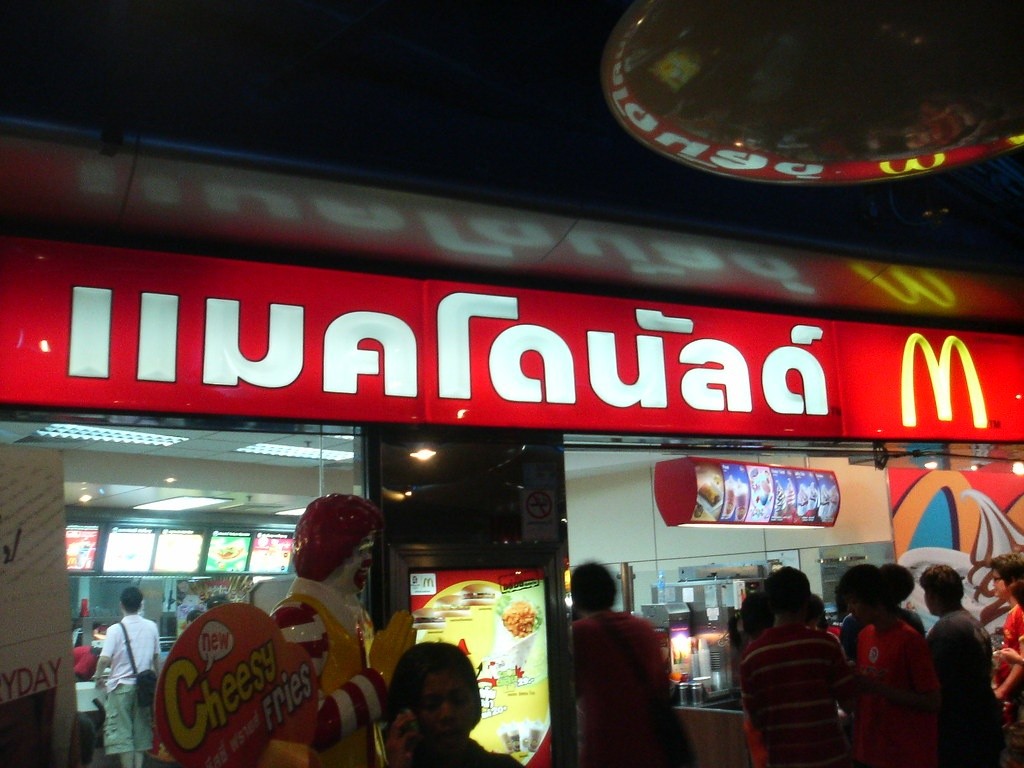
[271,495,418,768]
[383,642,527,768]
[72,554,1024,768]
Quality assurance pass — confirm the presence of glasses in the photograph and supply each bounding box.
[993,576,1002,582]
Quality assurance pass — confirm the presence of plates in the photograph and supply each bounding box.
[696,479,723,513]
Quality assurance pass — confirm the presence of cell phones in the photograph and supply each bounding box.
[398,709,419,745]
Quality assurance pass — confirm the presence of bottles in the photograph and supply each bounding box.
[679,683,689,705]
[658,575,666,604]
[692,683,703,706]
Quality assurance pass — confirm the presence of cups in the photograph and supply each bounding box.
[724,477,735,518]
[696,638,712,676]
[498,722,542,754]
[733,483,748,520]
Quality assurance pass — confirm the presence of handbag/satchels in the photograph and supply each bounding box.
[137,670,158,706]
